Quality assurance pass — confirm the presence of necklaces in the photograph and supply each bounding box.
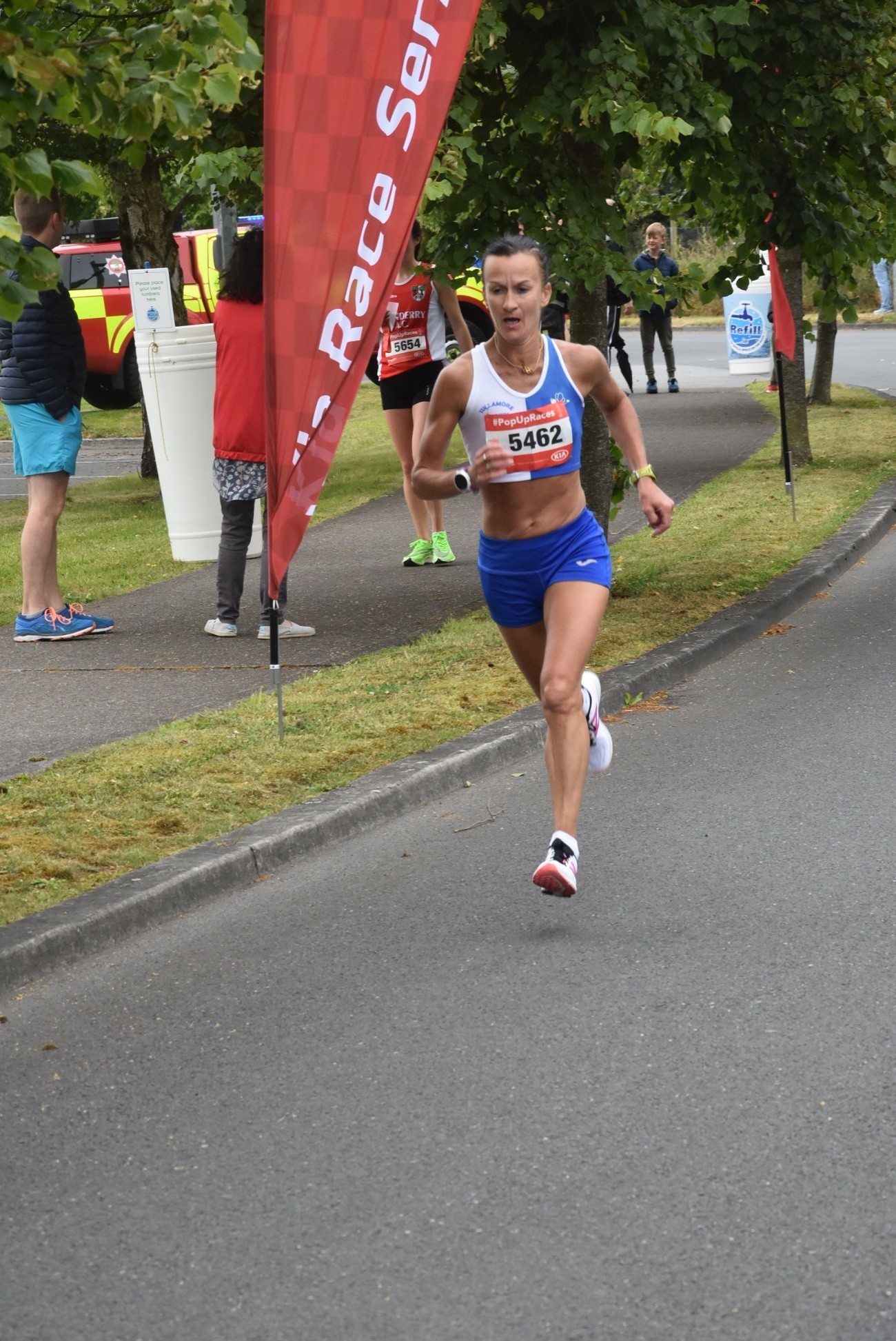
[493,332,544,374]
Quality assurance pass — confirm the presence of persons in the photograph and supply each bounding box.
[766,299,780,393]
[204,229,315,640]
[376,217,475,567]
[410,235,675,898]
[444,199,632,370]
[630,222,679,395]
[872,257,894,314]
[0,187,115,641]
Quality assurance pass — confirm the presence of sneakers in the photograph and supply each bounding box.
[767,385,779,393]
[532,838,579,898]
[646,379,659,393]
[401,538,433,566]
[204,617,237,637]
[432,532,456,563]
[667,378,679,393]
[13,607,96,642]
[55,603,114,632]
[257,621,316,639]
[580,671,613,774]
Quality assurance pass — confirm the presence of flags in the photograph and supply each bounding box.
[768,242,796,362]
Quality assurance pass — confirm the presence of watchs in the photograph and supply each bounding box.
[454,465,478,495]
[629,464,657,486]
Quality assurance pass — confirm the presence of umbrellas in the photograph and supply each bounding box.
[613,333,634,394]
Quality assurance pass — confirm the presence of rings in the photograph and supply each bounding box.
[483,453,487,460]
[487,462,490,472]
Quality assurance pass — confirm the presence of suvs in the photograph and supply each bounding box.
[52,215,495,408]
